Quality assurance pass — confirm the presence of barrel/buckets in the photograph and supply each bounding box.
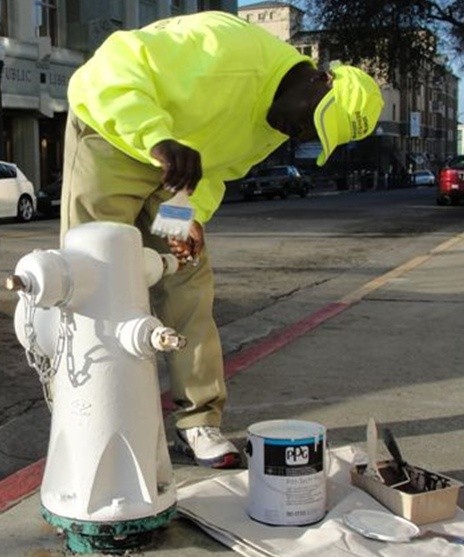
[247,419,327,526]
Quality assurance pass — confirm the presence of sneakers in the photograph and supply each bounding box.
[176,426,243,469]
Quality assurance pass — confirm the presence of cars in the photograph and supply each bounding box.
[0,161,37,223]
[436,156,464,205]
[240,165,310,201]
[411,170,435,184]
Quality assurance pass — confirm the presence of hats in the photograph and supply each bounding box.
[313,64,383,167]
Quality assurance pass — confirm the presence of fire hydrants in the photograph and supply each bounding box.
[5,221,191,550]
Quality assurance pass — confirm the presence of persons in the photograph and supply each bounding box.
[58,10,383,468]
